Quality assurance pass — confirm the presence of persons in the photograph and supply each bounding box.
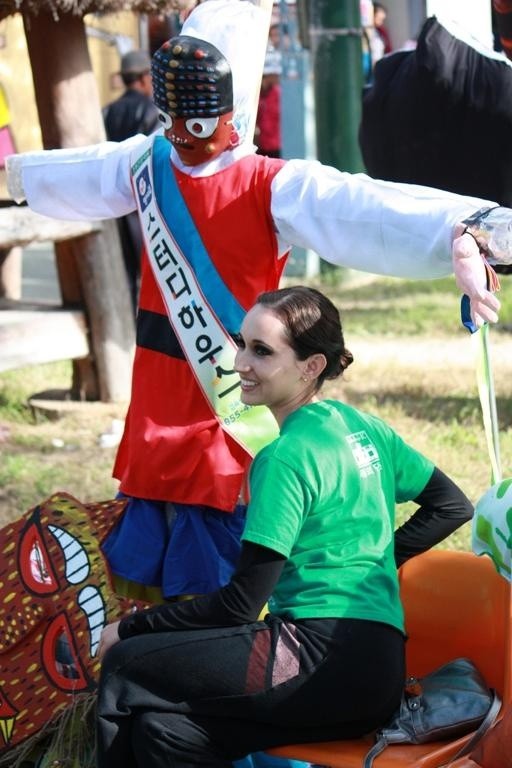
[4,6,500,593]
[371,2,394,55]
[89,286,476,767]
[255,22,291,159]
[104,33,163,143]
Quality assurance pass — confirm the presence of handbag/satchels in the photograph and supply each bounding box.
[374,656,496,744]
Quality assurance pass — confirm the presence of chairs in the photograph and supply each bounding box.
[264,547,510,768]
